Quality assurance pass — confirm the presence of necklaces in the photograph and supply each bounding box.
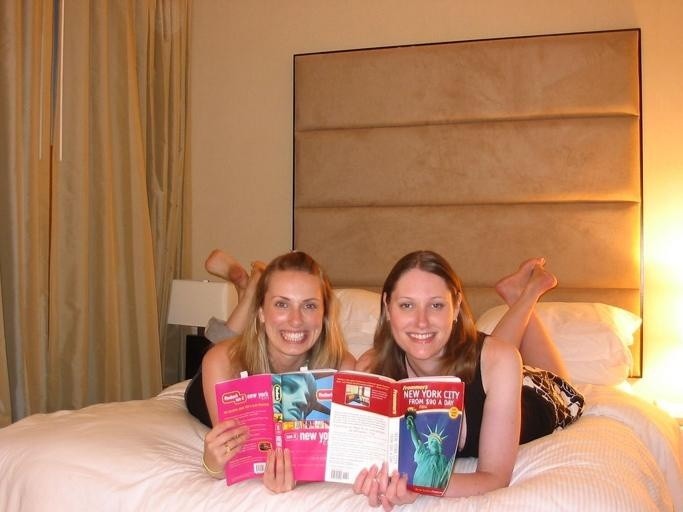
[405,357,453,377]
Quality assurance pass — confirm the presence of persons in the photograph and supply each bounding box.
[352,247,587,512]
[281,372,320,423]
[405,415,450,491]
[182,247,356,492]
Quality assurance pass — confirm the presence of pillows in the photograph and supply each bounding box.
[332,285,385,359]
[472,302,643,400]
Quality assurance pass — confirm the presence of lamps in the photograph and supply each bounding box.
[166,278,237,380]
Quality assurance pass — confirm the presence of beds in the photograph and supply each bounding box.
[0,377,682,512]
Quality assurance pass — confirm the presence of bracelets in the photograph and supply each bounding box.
[200,459,222,479]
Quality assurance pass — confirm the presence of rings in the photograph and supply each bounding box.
[378,492,385,497]
[224,443,230,455]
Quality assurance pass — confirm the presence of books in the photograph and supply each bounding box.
[214,368,339,486]
[322,371,466,498]
